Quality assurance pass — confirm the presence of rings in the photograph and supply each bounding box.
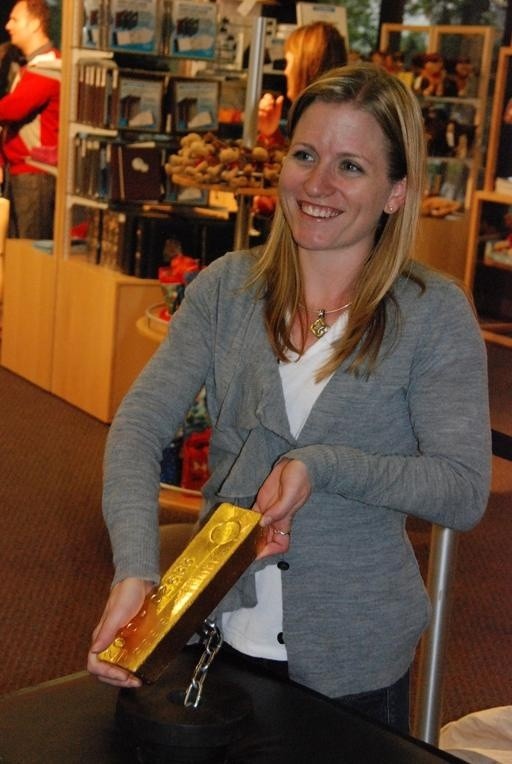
[273,529,290,536]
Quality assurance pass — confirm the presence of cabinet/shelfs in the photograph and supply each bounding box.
[7,1,512,466]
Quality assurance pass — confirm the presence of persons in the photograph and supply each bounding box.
[1,0,61,238]
[86,60,491,739]
[258,21,346,159]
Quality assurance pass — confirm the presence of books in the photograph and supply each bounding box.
[75,48,219,135]
[81,0,237,60]
[75,204,240,279]
[74,134,210,208]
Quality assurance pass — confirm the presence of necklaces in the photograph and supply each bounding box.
[299,298,351,336]
